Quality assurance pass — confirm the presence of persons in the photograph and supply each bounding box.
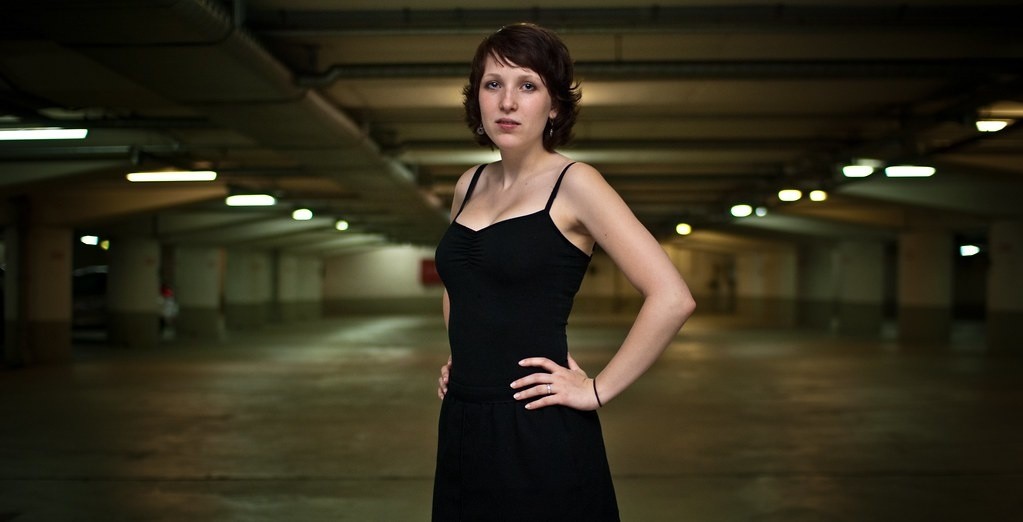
[430,22,697,522]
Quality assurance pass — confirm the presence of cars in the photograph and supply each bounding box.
[71,263,173,338]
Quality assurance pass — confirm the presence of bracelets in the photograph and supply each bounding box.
[593,379,603,408]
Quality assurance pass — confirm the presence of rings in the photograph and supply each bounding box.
[547,384,551,393]
